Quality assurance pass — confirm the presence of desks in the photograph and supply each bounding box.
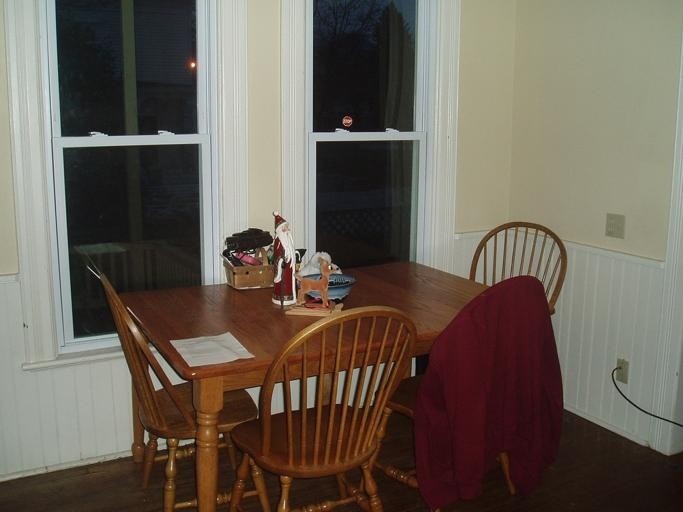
[116,262,558,508]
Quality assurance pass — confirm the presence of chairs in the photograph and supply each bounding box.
[227,304,420,511]
[371,276,567,509]
[376,222,567,422]
[88,264,272,510]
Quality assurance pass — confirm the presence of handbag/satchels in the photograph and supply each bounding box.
[225,228,273,251]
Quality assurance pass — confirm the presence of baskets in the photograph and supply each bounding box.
[223,247,301,290]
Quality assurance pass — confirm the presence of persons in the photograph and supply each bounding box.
[271,211,297,306]
